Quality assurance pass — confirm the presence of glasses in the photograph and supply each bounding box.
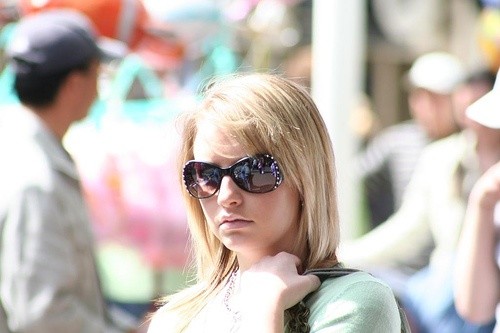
[182,151,284,200]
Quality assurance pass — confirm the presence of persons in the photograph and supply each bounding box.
[337,48,500,333]
[148,74,402,333]
[0,9,136,333]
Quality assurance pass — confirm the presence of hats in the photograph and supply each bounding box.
[465,66,500,131]
[7,8,127,79]
[403,51,469,95]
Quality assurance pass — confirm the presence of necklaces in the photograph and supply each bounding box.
[223,265,242,315]
